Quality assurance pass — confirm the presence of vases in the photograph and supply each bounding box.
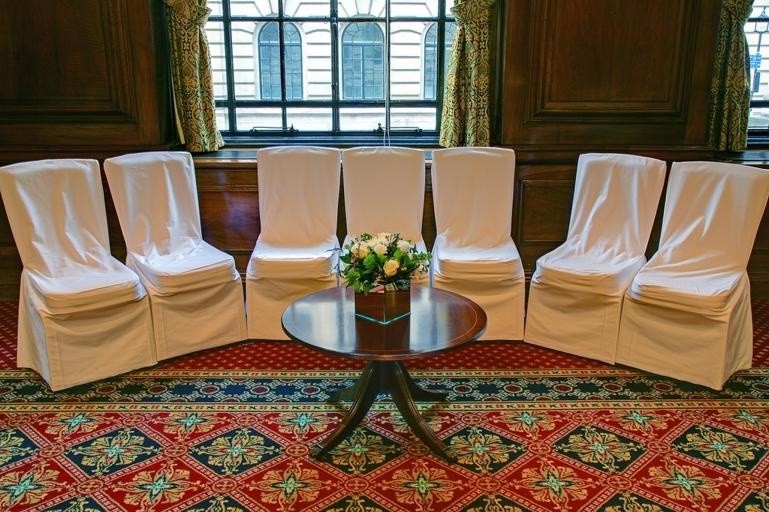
[354,284,410,324]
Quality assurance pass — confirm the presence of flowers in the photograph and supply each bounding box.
[328,231,433,294]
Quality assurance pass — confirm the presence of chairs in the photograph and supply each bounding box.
[524,153,667,366]
[339,146,430,287]
[103,151,247,362]
[614,161,769,391]
[432,146,526,341]
[247,147,341,341]
[0,159,159,392]
